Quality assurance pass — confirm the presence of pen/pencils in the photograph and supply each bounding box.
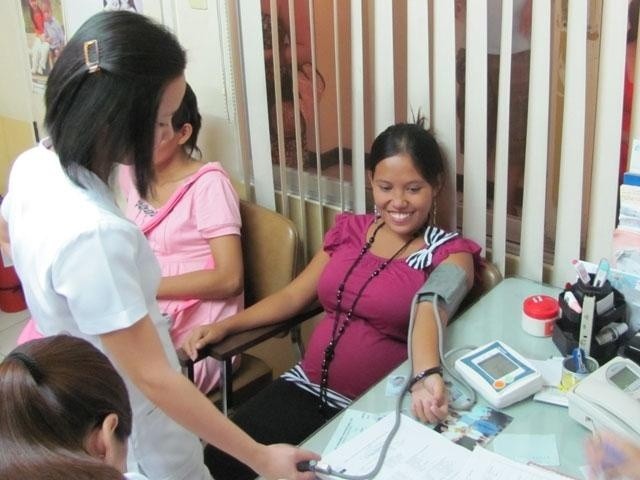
[527,461,578,480]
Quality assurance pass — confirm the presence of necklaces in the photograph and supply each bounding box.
[318,222,428,410]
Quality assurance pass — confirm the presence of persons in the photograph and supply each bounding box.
[181,104,481,480]
[0,10,321,480]
[434,399,512,451]
[41,0,65,70]
[20,0,50,75]
[16,84,244,397]
[0,333,147,480]
[262,13,325,171]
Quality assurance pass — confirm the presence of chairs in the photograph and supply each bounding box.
[181,199,305,414]
[207,253,505,423]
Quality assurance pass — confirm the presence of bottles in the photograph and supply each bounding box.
[521,294,562,337]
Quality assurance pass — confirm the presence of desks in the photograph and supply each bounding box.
[250,272,640,480]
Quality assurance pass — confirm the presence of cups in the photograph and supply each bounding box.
[559,353,599,395]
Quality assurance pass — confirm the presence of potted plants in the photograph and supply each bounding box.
[266,57,329,171]
